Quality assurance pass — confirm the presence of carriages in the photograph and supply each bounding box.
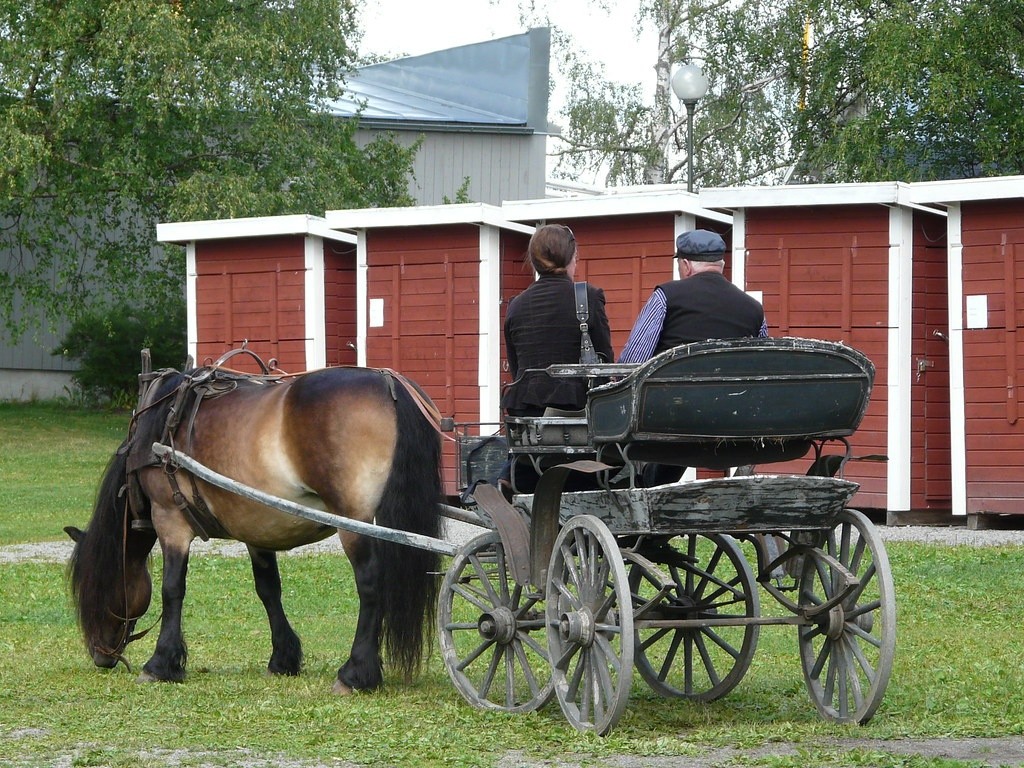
[61,336,894,741]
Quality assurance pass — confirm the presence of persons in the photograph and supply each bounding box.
[608,228,768,487]
[501,225,615,462]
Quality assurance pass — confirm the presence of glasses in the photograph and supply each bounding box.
[562,226,575,240]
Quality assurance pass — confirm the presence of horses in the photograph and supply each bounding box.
[63,368,444,695]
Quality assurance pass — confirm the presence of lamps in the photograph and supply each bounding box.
[672,66,708,192]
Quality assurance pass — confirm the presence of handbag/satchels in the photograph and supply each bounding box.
[573,280,610,388]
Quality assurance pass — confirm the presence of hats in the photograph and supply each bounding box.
[671,229,726,262]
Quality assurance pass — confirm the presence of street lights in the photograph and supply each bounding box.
[670,63,708,192]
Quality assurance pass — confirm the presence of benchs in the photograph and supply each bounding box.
[499,363,644,493]
[587,335,876,489]
